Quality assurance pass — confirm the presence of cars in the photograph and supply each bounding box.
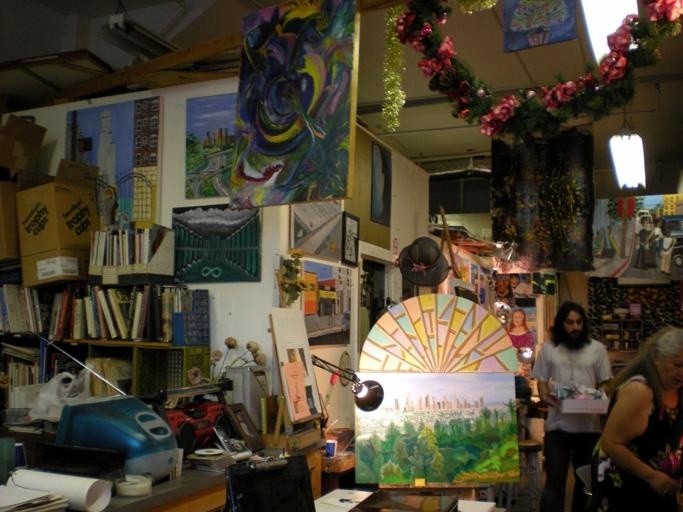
[635,209,683,267]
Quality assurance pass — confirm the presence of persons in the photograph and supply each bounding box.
[594,216,676,276]
[506,310,534,356]
[528,300,615,512]
[589,323,682,510]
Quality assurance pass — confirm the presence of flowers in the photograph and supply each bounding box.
[400,2,683,145]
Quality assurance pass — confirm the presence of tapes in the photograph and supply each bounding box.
[115,475,152,497]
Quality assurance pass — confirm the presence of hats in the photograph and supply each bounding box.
[397,236,448,286]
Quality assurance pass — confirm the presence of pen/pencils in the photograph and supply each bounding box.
[340,499,363,503]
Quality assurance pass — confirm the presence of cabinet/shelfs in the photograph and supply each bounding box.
[599,315,644,352]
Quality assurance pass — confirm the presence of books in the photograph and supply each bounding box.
[0,482,71,512]
[1,284,195,344]
[2,344,132,409]
[90,223,169,266]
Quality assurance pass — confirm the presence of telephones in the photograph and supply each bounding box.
[213,425,252,461]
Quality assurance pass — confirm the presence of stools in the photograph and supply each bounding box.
[495,438,545,512]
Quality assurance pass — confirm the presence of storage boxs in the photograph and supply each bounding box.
[1,109,105,290]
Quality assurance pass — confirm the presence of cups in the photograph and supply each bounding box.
[14,442,27,470]
[325,440,337,459]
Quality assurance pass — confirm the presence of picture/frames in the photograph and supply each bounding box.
[368,140,392,227]
[340,210,362,268]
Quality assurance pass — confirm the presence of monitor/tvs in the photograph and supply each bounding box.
[226,455,315,512]
